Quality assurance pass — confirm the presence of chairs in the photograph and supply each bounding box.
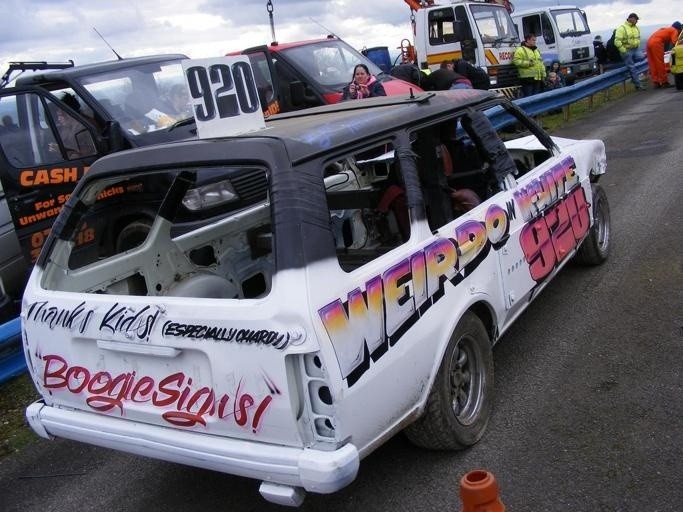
[386,146,482,244]
[43,101,68,144]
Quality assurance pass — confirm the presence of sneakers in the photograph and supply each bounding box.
[634,81,675,92]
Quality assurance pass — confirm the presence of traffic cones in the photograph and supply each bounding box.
[458,469,505,512]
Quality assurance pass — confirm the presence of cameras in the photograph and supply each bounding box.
[354,84,361,90]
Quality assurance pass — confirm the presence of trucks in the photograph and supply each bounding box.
[0,49,274,292]
[506,5,601,88]
[389,0,530,101]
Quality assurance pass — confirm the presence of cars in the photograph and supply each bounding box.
[669,29,683,91]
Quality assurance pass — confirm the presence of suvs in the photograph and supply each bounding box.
[15,81,614,510]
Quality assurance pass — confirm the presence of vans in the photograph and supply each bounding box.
[224,31,426,113]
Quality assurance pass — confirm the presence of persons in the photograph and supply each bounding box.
[605,29,623,63]
[546,58,568,88]
[645,21,682,89]
[390,58,490,92]
[380,115,481,182]
[341,63,386,100]
[613,13,647,90]
[159,83,190,120]
[546,72,560,90]
[593,35,605,65]
[511,32,545,97]
[45,99,95,158]
